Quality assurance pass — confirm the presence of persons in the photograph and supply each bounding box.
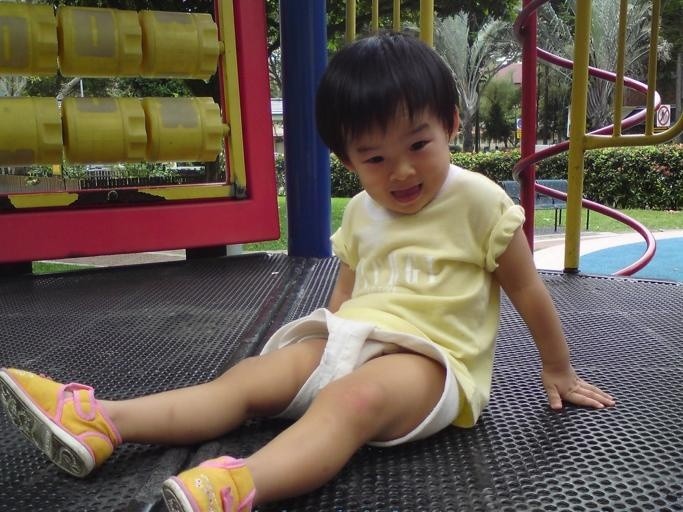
[0,27,615,512]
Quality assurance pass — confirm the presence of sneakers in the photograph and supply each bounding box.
[0,368,121,479]
[162,456,256,512]
